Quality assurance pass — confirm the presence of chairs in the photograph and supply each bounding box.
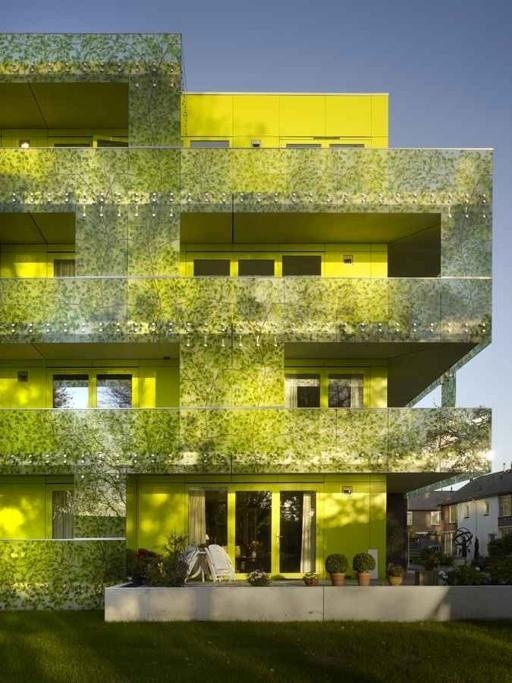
[180,543,235,584]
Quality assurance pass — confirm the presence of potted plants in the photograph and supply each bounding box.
[303,553,473,585]
